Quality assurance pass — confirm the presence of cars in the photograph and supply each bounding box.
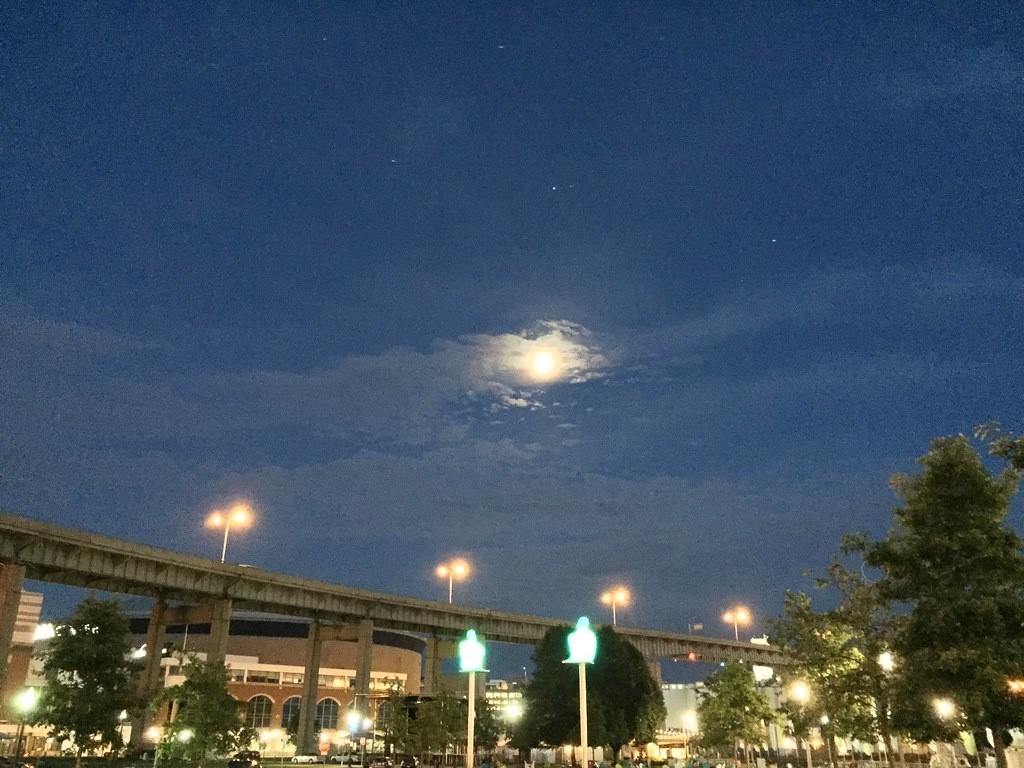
[291,752,318,764]
[0,756,34,768]
[330,751,358,763]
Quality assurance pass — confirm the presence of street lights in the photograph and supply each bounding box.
[16,686,37,755]
[208,506,252,564]
[435,558,471,602]
[602,588,632,626]
[457,631,490,768]
[723,609,751,641]
[562,616,597,768]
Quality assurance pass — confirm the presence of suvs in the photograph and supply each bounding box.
[233,751,261,762]
